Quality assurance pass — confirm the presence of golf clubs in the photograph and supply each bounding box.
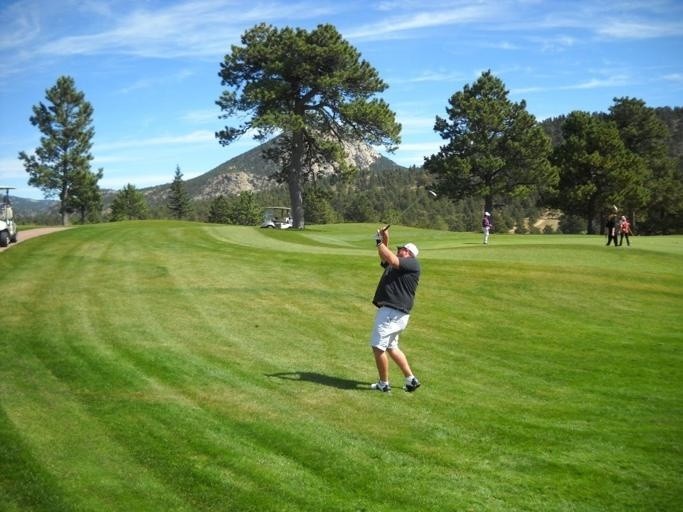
[383,190,436,232]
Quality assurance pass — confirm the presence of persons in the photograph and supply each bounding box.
[480,212,493,245]
[605,205,617,246]
[369,227,421,392]
[618,216,630,246]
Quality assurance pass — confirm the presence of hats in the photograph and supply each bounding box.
[396,242,419,258]
[484,212,491,216]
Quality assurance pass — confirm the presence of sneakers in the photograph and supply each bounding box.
[370,380,391,392]
[403,377,421,392]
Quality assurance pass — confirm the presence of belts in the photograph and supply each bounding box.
[378,304,408,314]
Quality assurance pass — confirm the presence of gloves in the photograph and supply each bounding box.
[375,229,383,247]
